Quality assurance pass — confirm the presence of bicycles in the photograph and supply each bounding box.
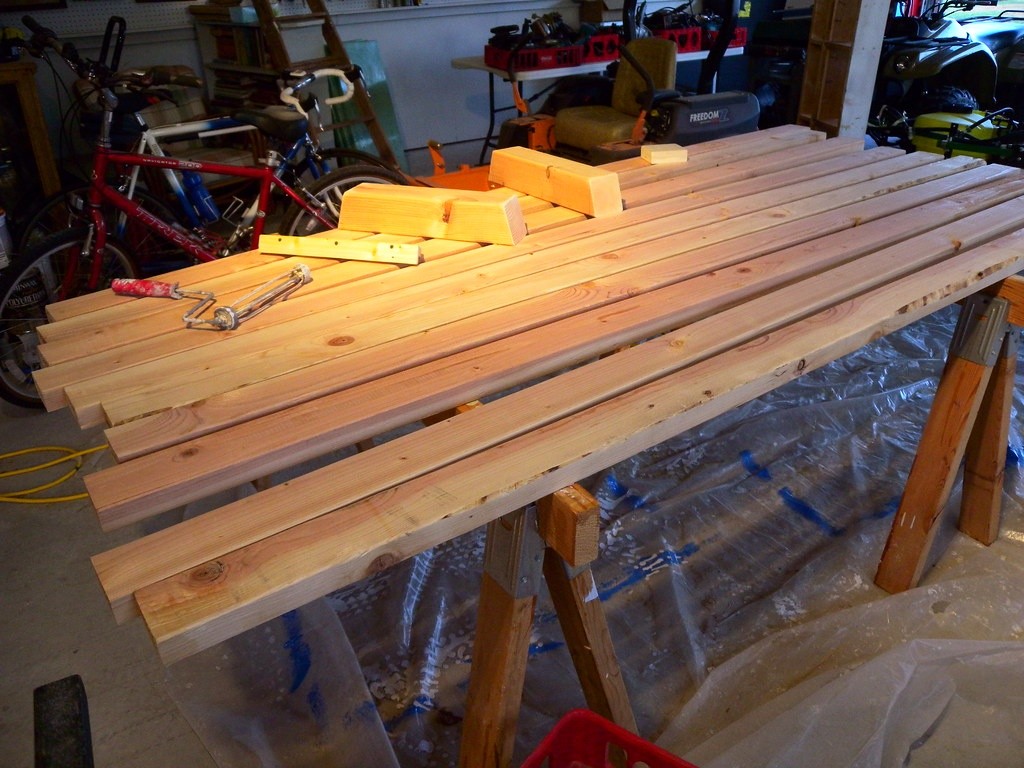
[0,14,408,409]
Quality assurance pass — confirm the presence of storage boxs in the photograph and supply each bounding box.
[484,26,748,73]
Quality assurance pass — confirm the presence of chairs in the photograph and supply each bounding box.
[73,63,267,197]
[556,38,677,149]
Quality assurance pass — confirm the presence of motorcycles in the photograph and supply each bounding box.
[885,0,1023,108]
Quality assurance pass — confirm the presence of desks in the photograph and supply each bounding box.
[31,124,1024,768]
[452,47,744,166]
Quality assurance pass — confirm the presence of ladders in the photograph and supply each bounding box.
[252,0,403,173]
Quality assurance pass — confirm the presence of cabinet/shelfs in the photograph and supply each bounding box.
[187,13,339,169]
[796,0,891,139]
